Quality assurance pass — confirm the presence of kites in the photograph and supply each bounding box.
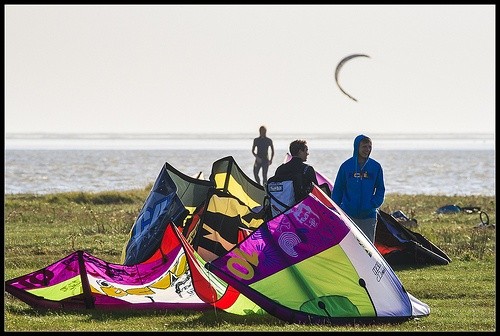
[11,152,453,323]
[335,53,372,101]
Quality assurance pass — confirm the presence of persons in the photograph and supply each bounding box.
[275,139,319,204]
[251,126,274,185]
[332,135,386,246]
